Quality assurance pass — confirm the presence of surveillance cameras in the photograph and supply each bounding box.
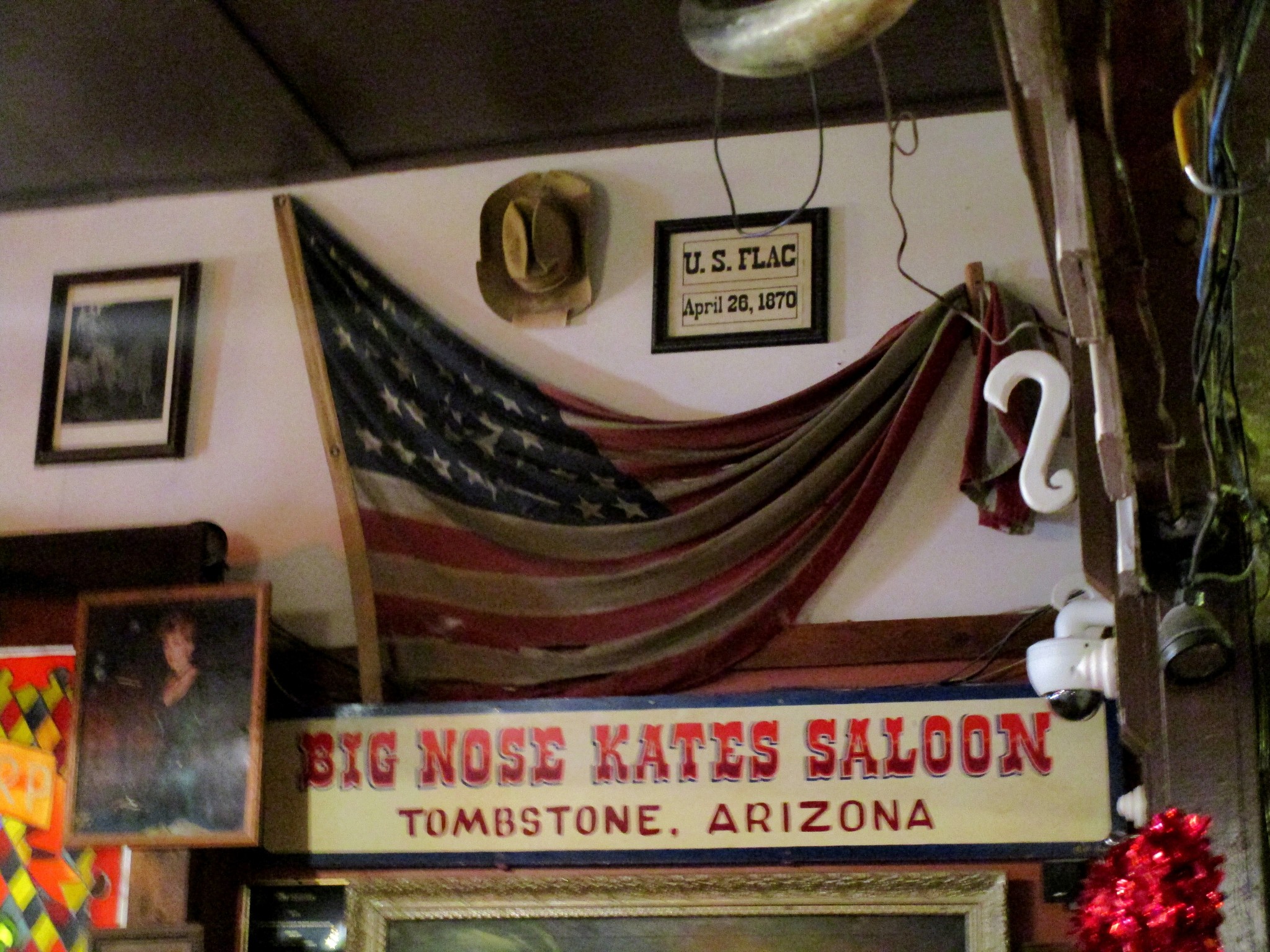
[1026,637,1110,723]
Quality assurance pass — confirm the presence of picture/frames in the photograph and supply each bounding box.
[35,261,202,466]
[64,581,273,847]
[652,208,830,355]
[349,871,1007,952]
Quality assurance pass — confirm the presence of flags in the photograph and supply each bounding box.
[272,192,1061,711]
